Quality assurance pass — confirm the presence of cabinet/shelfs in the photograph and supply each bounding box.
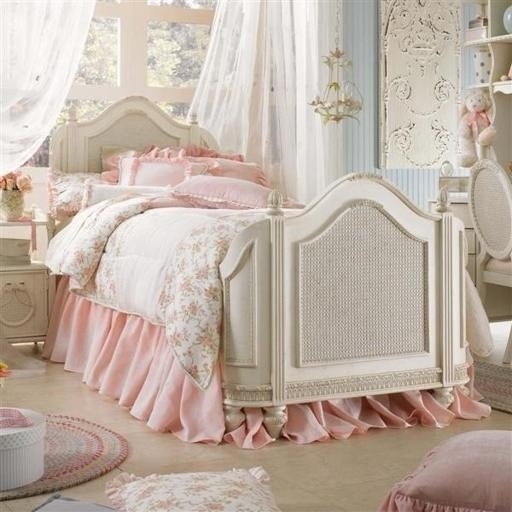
[424,1,511,329]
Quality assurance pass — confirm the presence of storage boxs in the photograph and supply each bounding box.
[463,28,489,43]
[1,403,47,492]
[468,17,489,28]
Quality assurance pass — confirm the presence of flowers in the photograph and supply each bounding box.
[310,46,364,128]
[1,168,35,193]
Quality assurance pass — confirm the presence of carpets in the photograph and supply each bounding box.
[1,407,131,502]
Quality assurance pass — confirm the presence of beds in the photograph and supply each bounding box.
[44,91,472,447]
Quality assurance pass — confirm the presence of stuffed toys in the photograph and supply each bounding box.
[458,90,495,168]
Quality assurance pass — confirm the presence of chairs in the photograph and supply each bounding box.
[464,157,510,365]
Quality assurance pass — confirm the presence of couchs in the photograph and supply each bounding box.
[375,428,512,510]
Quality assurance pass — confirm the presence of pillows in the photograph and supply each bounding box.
[105,464,284,512]
[47,142,287,221]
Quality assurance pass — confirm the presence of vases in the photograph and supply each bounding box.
[1,191,28,221]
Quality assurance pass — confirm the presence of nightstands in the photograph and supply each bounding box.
[0,216,54,354]
